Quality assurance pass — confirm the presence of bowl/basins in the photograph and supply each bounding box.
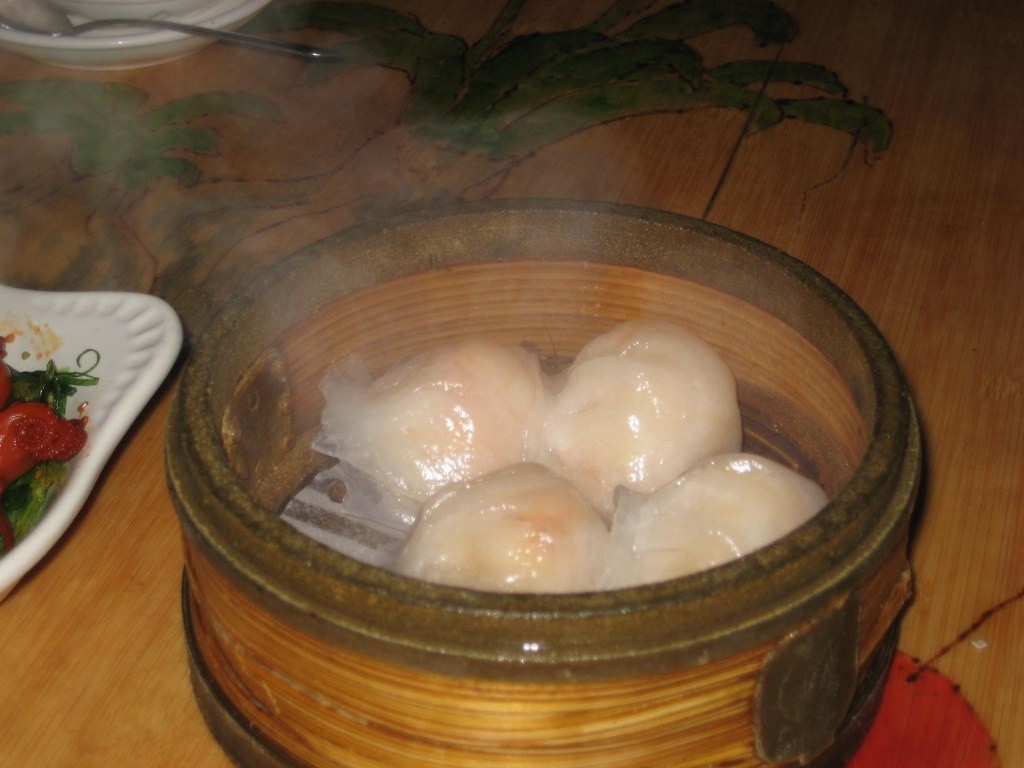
[0,0,271,72]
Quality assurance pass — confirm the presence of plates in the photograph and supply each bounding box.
[0,283,184,601]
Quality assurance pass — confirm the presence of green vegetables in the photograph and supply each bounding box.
[0,348,102,557]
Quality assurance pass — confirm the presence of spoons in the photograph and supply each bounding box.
[0,0,339,65]
